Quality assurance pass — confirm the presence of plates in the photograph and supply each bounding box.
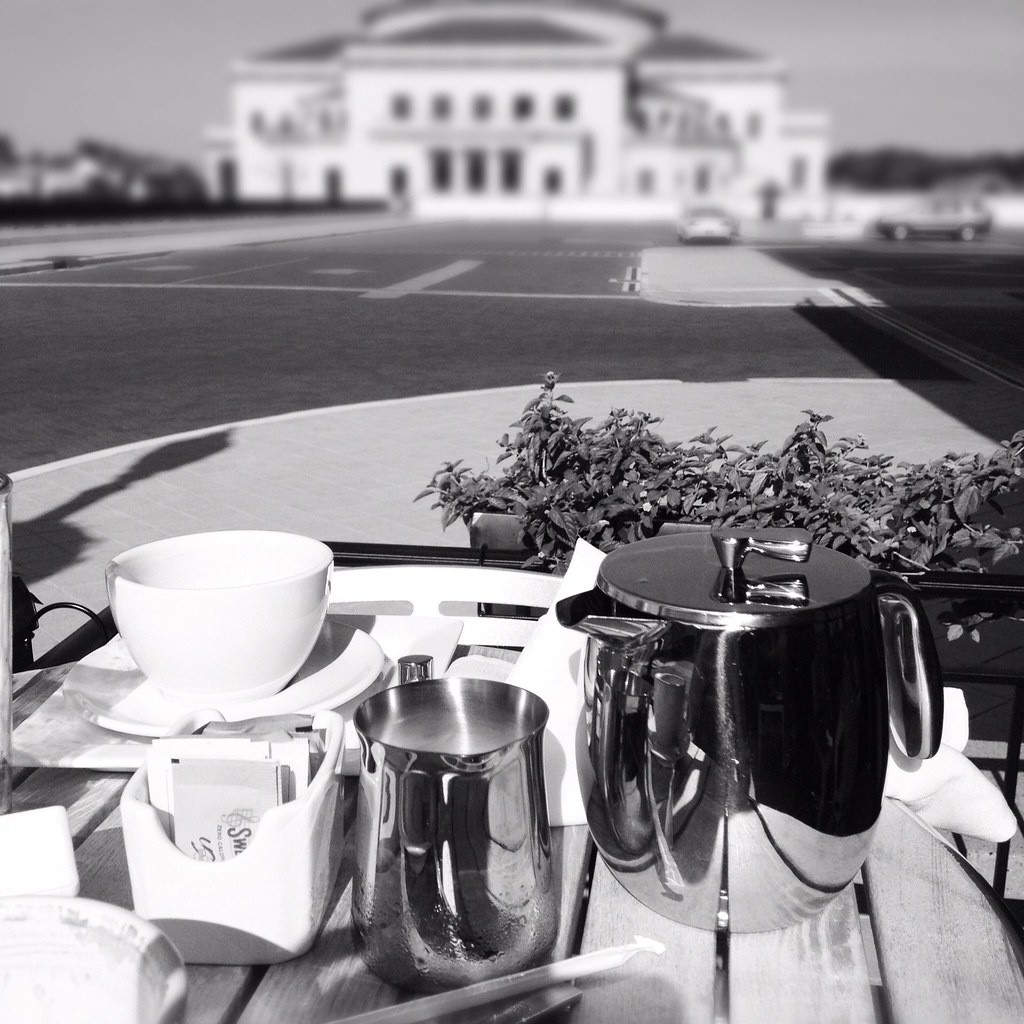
[10,614,464,773]
[62,620,384,738]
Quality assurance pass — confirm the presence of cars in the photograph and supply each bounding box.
[674,203,732,244]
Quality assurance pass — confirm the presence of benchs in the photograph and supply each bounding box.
[470,510,1024,900]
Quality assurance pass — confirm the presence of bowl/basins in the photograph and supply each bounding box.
[105,531,334,711]
[7,894,192,1023]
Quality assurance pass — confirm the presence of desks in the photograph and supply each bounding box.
[0,659,1024,1024]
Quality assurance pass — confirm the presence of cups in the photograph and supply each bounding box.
[354,656,562,993]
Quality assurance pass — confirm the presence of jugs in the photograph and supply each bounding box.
[556,525,942,922]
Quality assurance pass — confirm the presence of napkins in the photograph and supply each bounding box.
[501,537,1017,843]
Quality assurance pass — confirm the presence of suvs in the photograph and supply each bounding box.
[876,190,993,243]
